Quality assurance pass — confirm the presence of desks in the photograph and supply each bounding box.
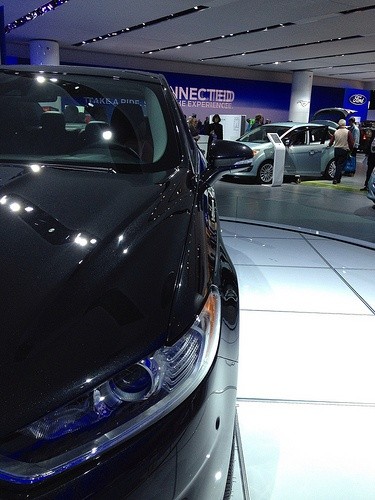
[194,134,209,162]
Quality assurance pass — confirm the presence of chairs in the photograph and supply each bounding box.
[111,104,154,162]
[74,121,109,156]
[34,111,72,154]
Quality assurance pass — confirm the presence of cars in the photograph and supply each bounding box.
[0,61,256,500]
[310,108,375,154]
[222,120,339,185]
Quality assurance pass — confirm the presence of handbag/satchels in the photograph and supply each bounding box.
[344,151,356,171]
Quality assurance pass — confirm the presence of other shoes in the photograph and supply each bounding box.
[351,173,354,177]
[333,179,337,184]
[348,173,351,176]
[337,179,340,183]
[360,186,369,191]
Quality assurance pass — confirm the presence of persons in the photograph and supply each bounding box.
[348,117,360,176]
[204,114,223,140]
[360,133,375,191]
[186,114,205,142]
[249,114,263,142]
[265,119,269,124]
[328,119,354,185]
[246,118,255,129]
[261,117,264,125]
